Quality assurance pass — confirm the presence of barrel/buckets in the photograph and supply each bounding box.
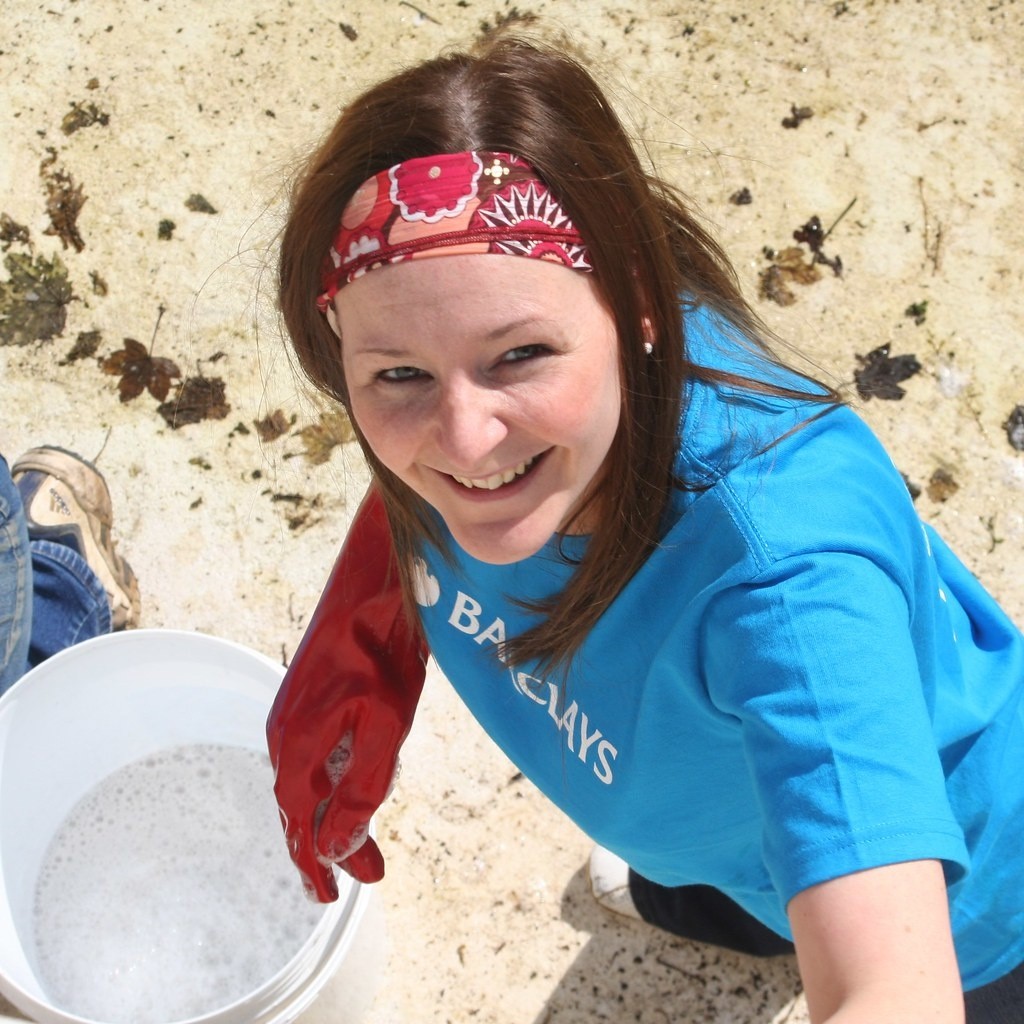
[1,628,360,1024]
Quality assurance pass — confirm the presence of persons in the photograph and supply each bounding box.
[265,34,1024,1024]
[0,446,140,697]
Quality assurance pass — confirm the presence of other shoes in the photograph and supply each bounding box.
[588,846,644,921]
[12,446,141,631]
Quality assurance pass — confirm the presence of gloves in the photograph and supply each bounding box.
[265,475,429,904]
